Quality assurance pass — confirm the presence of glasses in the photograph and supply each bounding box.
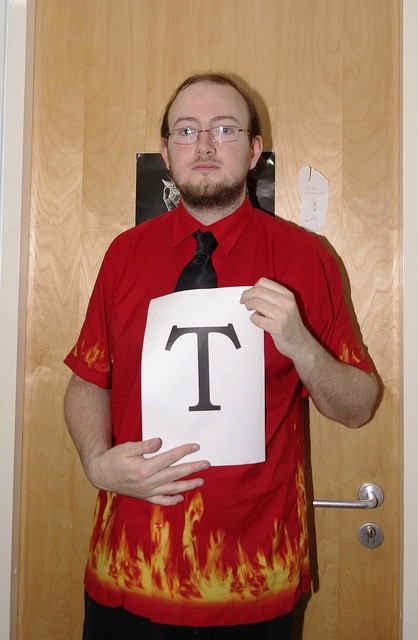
[168,125,250,144]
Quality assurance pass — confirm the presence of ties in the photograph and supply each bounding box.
[174,228,221,291]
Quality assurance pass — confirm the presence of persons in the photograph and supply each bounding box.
[64,73,384,640]
